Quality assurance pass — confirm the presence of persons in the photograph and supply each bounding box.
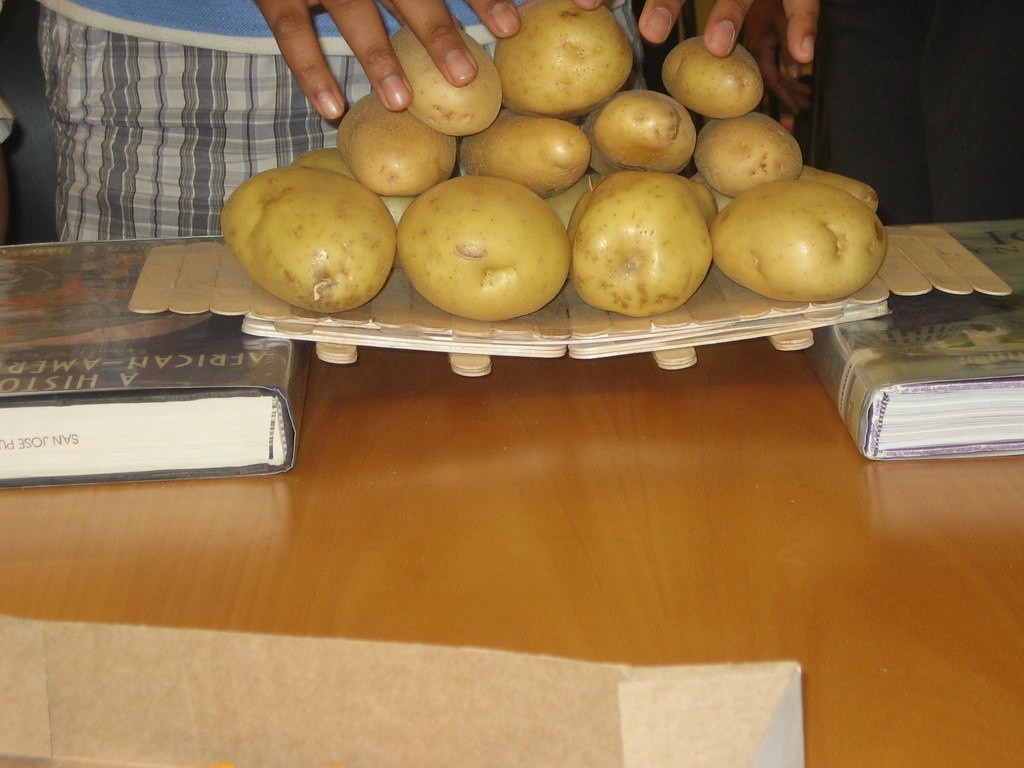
[745,0,1023,224]
[36,0,826,244]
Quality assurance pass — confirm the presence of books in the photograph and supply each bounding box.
[806,219,1024,461]
[0,236,306,490]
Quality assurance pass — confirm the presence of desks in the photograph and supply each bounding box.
[0,336,1023,767]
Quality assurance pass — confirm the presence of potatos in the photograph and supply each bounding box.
[221,0,888,323]
